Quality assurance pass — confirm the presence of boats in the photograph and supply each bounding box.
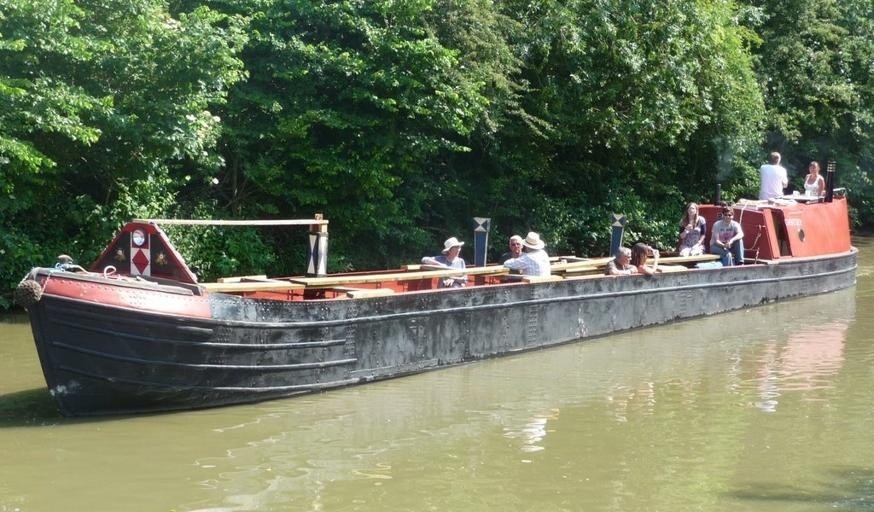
[11,188,864,419]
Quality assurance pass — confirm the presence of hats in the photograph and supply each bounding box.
[520,232,544,249]
[440,237,464,254]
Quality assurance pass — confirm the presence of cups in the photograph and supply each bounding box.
[805,190,811,199]
[792,191,800,199]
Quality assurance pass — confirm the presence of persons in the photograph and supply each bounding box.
[802,160,825,205]
[419,235,467,291]
[604,246,637,277]
[757,151,789,201]
[708,206,745,267]
[502,231,551,276]
[629,242,662,277]
[675,201,707,256]
[492,234,526,284]
